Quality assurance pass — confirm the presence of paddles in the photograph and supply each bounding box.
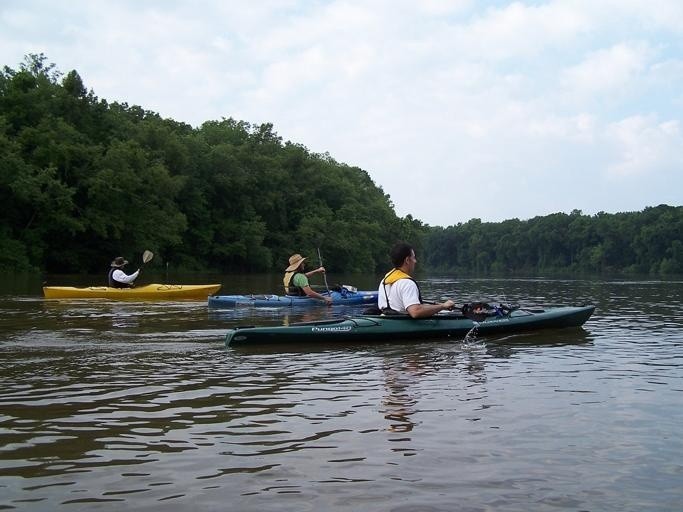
[316,248,331,298]
[132,251,154,284]
[420,299,489,321]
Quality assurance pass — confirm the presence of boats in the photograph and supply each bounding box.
[210,306,369,325]
[224,304,596,347]
[41,283,221,302]
[206,286,378,308]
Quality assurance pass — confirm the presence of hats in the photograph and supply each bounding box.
[285,254,306,272]
[111,257,128,267]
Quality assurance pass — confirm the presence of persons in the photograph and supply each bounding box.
[109,256,140,288]
[283,254,333,306]
[378,245,455,318]
